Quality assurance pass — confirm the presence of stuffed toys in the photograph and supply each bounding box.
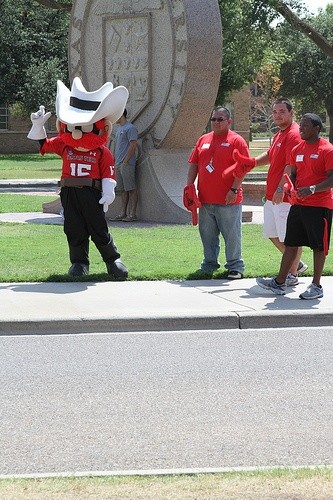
[27,76,129,278]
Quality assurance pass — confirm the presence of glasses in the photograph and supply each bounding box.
[210,117,228,122]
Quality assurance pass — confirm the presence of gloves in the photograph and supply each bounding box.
[27,104,52,141]
[99,176,118,213]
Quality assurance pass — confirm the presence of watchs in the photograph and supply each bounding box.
[310,186,315,194]
[230,188,238,194]
[276,187,283,193]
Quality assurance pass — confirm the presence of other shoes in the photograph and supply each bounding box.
[198,267,219,272]
[122,215,136,222]
[227,271,242,279]
[109,213,127,221]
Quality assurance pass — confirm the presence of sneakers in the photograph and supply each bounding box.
[297,260,308,273]
[299,283,323,300]
[286,272,299,286]
[256,276,287,295]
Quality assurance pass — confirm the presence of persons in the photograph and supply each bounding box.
[109,109,138,222]
[183,106,250,281]
[222,97,308,286]
[255,112,333,300]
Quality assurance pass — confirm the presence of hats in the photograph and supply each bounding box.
[54,74,129,127]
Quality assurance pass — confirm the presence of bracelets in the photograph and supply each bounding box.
[123,159,127,164]
